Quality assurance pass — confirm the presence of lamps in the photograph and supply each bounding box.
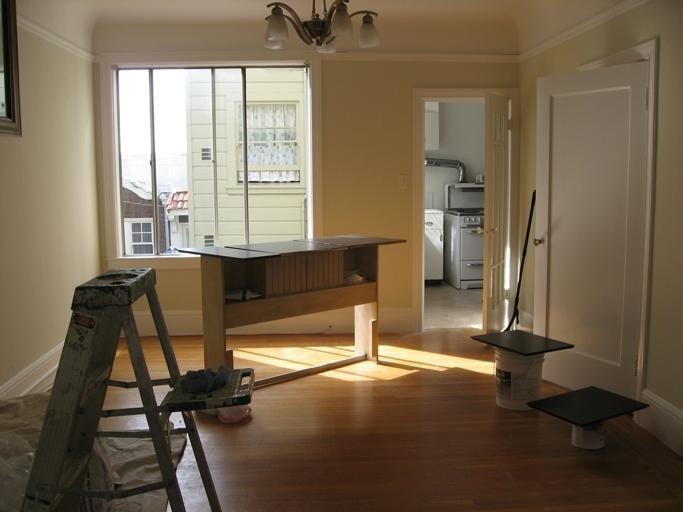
[262,0,379,55]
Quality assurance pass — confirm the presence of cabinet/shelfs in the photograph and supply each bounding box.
[425,211,444,284]
[445,215,484,290]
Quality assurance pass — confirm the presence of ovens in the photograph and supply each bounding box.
[460,228,483,281]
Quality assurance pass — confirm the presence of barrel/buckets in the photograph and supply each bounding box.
[495,350,546,411]
[572,424,607,449]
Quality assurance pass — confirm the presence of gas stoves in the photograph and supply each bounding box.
[447,208,484,215]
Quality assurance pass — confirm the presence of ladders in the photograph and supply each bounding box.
[21,268,254,512]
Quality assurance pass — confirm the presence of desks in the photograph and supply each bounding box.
[175,233,407,393]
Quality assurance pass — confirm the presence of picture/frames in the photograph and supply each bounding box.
[0,1,23,134]
